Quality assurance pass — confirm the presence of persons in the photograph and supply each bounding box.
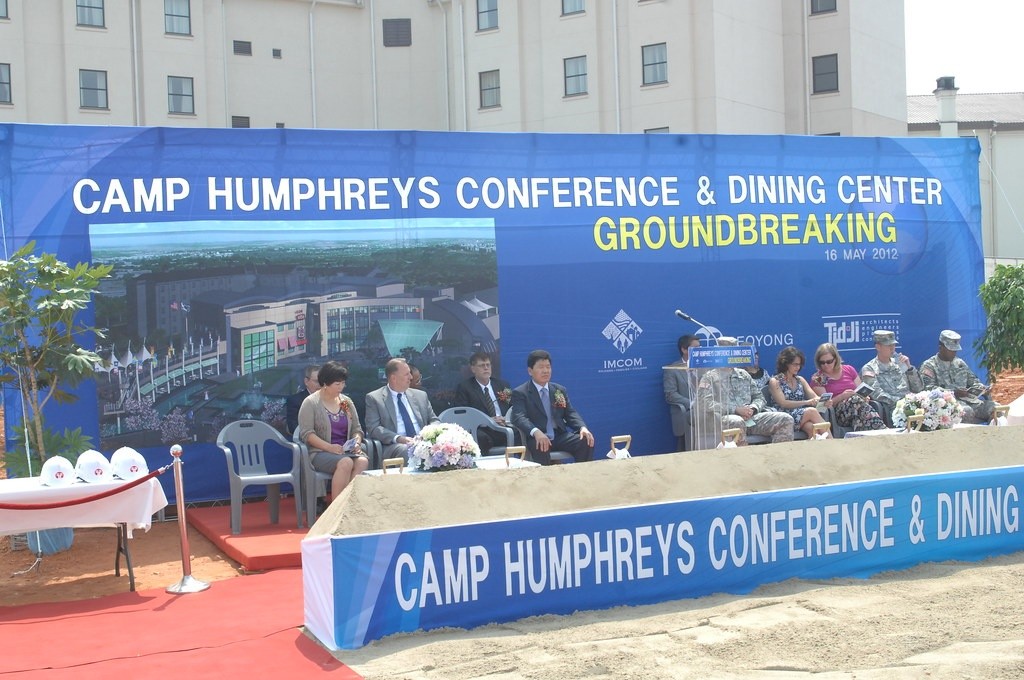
[286,361,369,504]
[407,363,428,393]
[512,350,595,466]
[364,358,441,469]
[739,342,770,390]
[810,343,889,431]
[663,335,702,452]
[455,352,523,459]
[695,336,794,447]
[862,329,925,412]
[768,346,833,440]
[919,330,1004,423]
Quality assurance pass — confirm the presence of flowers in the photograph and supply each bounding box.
[889,387,966,432]
[497,387,511,404]
[341,398,353,418]
[407,421,482,471]
[551,389,567,408]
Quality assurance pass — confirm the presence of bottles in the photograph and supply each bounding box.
[892,351,908,372]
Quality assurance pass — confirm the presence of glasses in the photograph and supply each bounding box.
[791,363,800,368]
[305,376,318,384]
[476,363,492,368]
[817,356,835,364]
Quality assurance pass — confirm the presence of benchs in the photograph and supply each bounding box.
[881,392,993,429]
[685,399,830,451]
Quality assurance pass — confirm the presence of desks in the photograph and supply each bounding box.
[0,468,170,592]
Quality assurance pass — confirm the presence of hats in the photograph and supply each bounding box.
[939,329,963,351]
[717,337,738,345]
[873,330,899,346]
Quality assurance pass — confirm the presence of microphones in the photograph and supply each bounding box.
[674,309,719,347]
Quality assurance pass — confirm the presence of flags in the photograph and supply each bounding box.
[169,301,178,310]
[181,303,190,312]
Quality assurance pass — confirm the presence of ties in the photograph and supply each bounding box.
[397,393,417,438]
[484,387,496,417]
[541,388,555,441]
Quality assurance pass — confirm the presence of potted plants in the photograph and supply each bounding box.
[0,246,110,557]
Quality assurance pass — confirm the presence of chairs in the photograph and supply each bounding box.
[504,406,574,462]
[827,400,884,438]
[667,399,687,452]
[291,424,374,528]
[438,407,516,459]
[217,420,304,535]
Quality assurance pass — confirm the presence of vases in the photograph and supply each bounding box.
[913,416,942,431]
[436,459,456,471]
